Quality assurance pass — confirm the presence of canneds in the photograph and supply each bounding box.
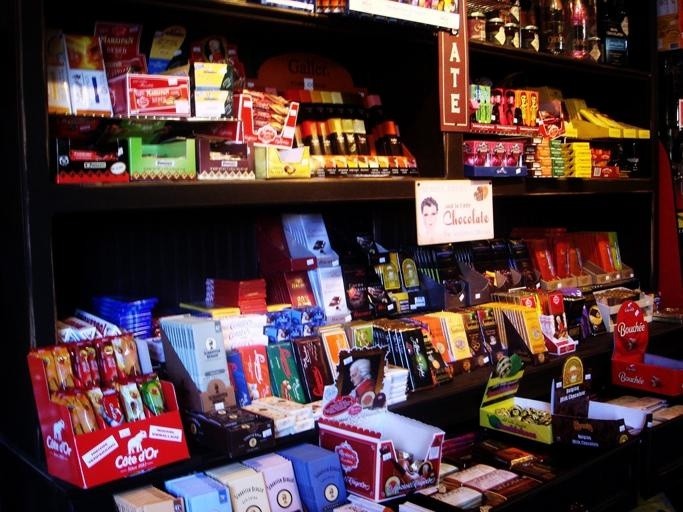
[467,12,540,52]
[587,36,602,63]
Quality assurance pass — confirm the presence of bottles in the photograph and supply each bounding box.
[540,1,565,55]
[565,1,590,60]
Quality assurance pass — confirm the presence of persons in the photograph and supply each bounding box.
[491,90,538,127]
[346,359,375,401]
[421,197,438,240]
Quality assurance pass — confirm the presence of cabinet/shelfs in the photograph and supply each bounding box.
[0,1,682,510]
